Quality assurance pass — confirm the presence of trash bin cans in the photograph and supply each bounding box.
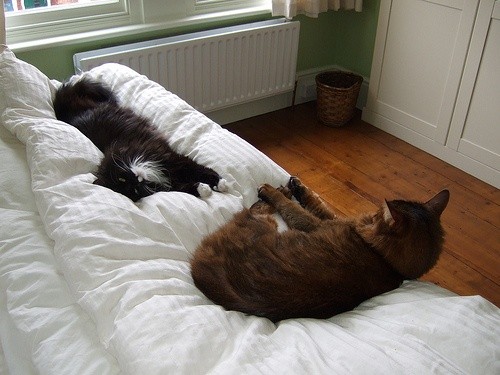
[315,70,364,128]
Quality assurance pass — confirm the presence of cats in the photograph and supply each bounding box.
[53,79,229,202]
[191,175,451,322]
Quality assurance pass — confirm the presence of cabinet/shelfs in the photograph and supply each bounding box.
[361,0,500,190]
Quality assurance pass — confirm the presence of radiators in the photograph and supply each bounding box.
[73,18,300,114]
